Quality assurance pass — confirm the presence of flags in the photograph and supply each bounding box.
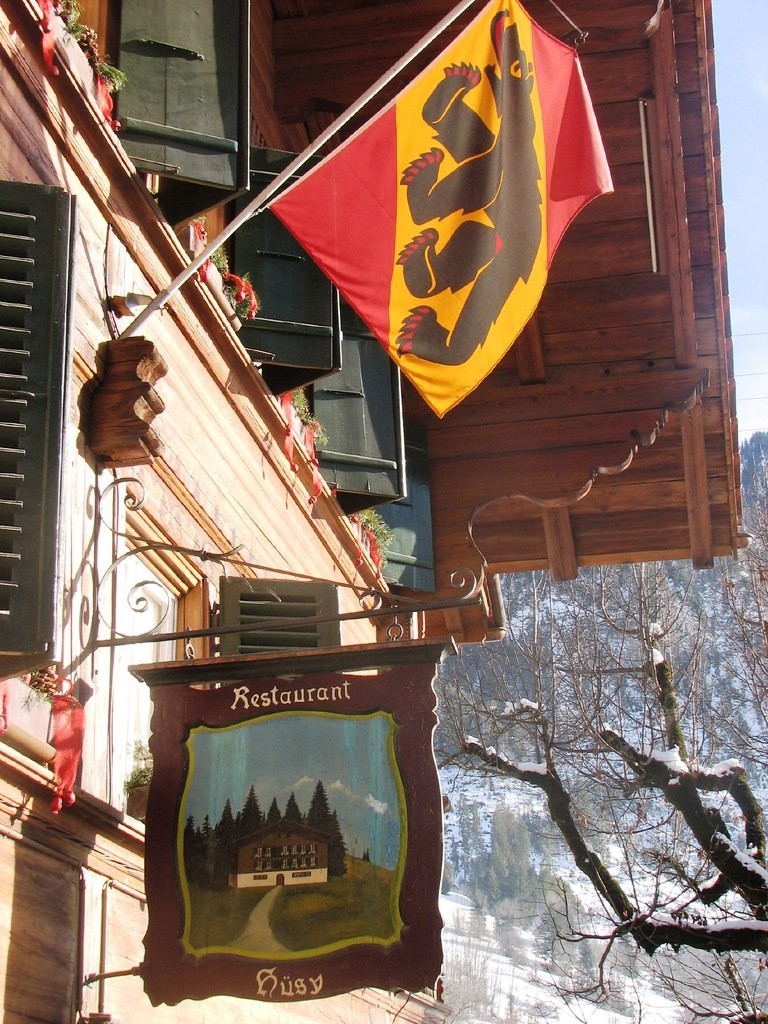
[262,0,615,421]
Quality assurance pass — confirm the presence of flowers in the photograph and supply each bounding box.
[149,187,262,322]
[48,0,129,94]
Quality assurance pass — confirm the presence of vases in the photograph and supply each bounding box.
[178,226,242,330]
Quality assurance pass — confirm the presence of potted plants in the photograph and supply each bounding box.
[349,506,397,570]
[281,386,331,467]
[123,738,154,824]
